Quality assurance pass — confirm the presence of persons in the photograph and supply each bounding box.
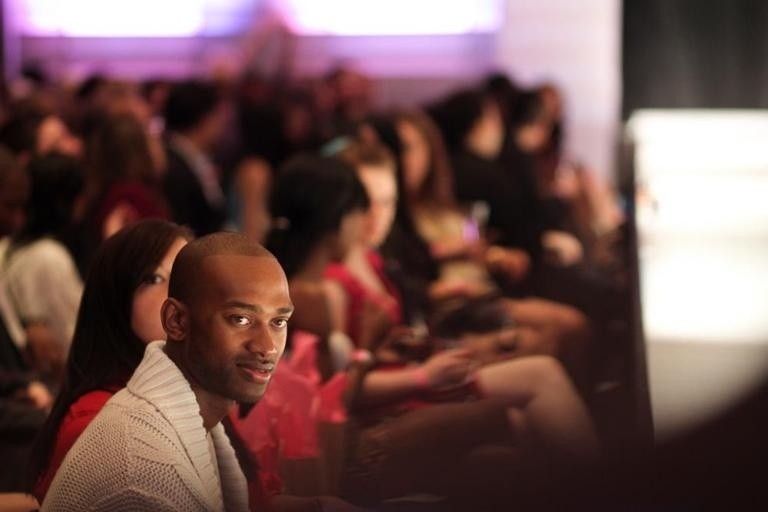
[228,54,611,512]
[73,74,271,242]
[1,56,107,492]
[37,219,187,497]
[41,230,294,512]
